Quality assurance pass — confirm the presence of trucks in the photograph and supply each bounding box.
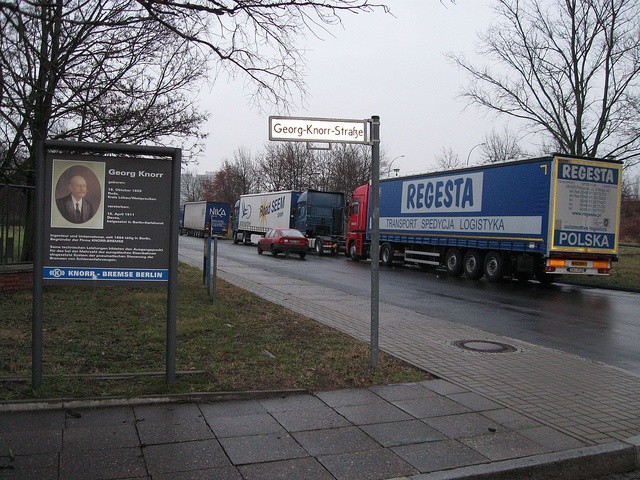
[233,190,303,246]
[346,154,623,284]
[179,201,230,239]
[295,189,346,255]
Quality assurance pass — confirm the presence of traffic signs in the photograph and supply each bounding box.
[269,117,369,142]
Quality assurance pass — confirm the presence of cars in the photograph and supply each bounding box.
[257,228,309,259]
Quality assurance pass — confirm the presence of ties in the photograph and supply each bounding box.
[75,202,81,223]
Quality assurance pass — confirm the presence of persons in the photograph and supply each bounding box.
[56,175,95,224]
